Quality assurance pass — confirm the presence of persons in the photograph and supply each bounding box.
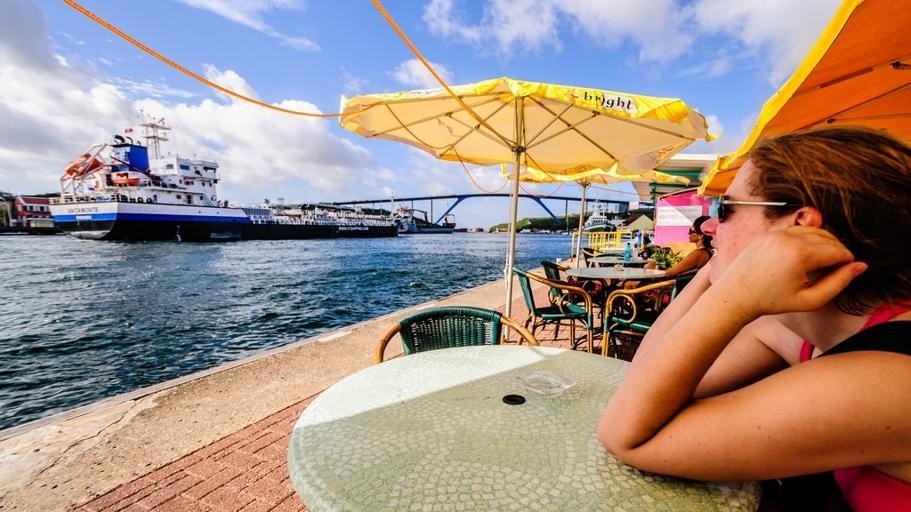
[665,215,714,280]
[596,124,911,511]
[628,230,651,248]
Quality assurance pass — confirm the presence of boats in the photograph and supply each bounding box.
[389,207,456,234]
[0,195,62,236]
[111,176,142,185]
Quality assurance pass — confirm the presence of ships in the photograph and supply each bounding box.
[48,107,400,241]
[583,193,617,234]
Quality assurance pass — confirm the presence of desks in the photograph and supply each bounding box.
[286,343,762,512]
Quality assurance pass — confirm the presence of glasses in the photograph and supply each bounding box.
[717,195,792,224]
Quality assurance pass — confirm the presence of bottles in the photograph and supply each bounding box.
[625,242,632,261]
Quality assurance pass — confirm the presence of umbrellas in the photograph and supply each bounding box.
[334,77,717,341]
[496,155,694,271]
[696,0,911,205]
